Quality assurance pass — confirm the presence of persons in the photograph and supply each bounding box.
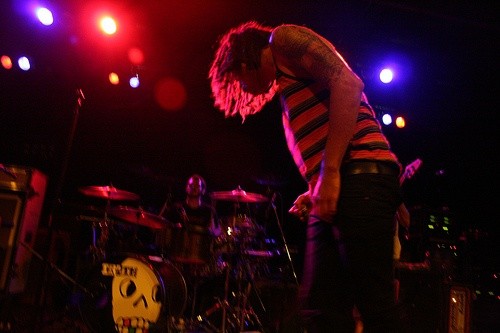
[208,18,402,331]
[392,198,410,304]
[161,173,219,232]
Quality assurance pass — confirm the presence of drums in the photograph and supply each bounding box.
[79,253,187,333]
[168,223,211,264]
[214,215,266,278]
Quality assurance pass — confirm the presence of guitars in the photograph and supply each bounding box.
[399,159,423,185]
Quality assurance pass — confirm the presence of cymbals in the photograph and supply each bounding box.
[210,185,268,203]
[77,186,141,200]
[110,205,169,229]
